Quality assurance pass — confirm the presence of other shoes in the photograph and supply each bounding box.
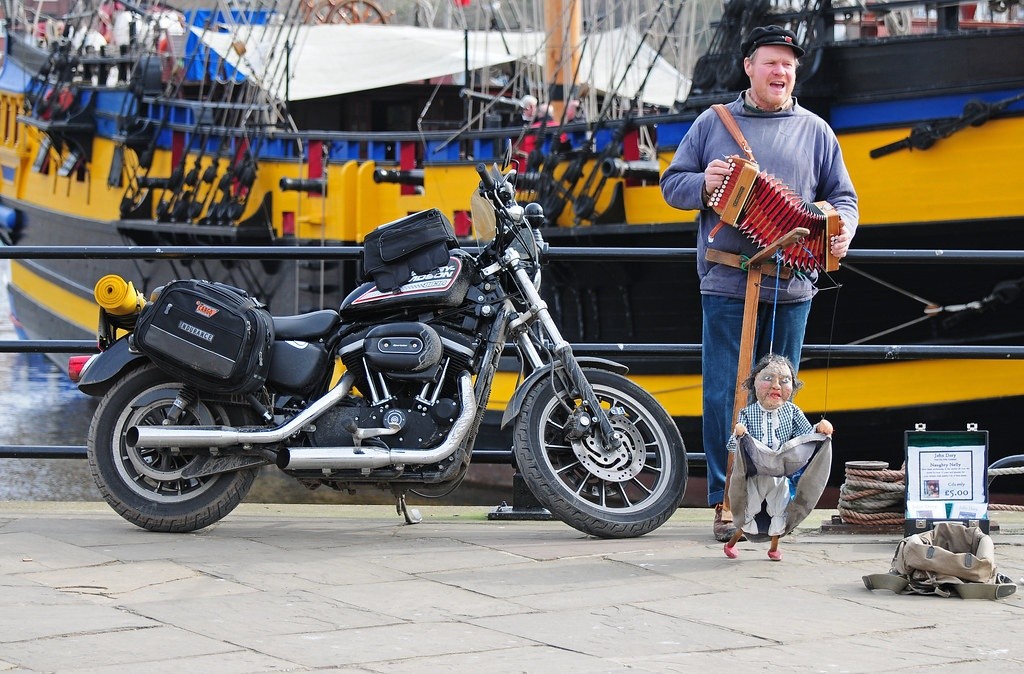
[755,515,770,534]
[713,503,748,542]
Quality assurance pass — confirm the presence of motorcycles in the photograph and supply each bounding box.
[69,137,689,541]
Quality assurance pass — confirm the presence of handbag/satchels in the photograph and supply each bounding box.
[358,206,460,294]
[134,280,275,395]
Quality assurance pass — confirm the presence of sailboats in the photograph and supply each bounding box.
[0,0,1024,485]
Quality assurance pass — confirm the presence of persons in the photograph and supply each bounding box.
[723,355,834,560]
[504,95,587,158]
[660,25,859,542]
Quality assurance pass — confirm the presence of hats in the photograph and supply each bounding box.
[518,95,538,108]
[740,24,805,59]
[539,103,554,116]
